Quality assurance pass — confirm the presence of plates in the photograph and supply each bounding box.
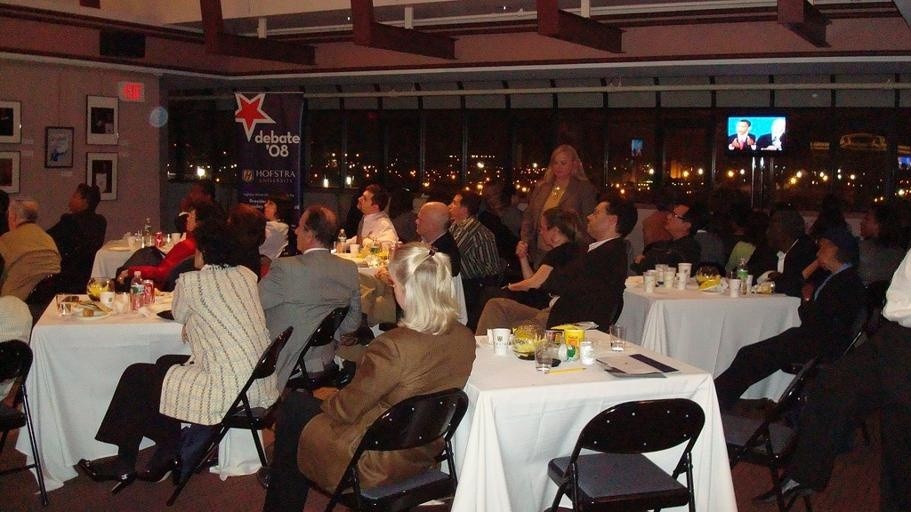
[74,310,110,320]
[109,246,131,252]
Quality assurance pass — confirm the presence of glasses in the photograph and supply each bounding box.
[672,211,685,220]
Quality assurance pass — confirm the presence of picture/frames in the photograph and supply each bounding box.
[86,94,120,146]
[0,100,22,144]
[0,151,21,194]
[86,153,118,201]
[45,125,74,167]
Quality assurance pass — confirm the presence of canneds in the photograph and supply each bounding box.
[155,232,163,249]
[143,279,155,304]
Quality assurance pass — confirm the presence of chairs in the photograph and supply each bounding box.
[322,388,470,511]
[166,325,284,507]
[289,306,349,401]
[0,340,50,505]
[540,397,706,512]
[714,357,823,511]
[782,326,870,377]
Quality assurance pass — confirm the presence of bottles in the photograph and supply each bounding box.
[130,271,144,310]
[144,216,152,232]
[337,229,347,254]
[369,236,381,269]
[735,257,748,295]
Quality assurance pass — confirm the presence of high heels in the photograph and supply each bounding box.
[138,457,181,484]
[74,459,137,494]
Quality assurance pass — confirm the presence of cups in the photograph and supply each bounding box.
[349,243,360,255]
[609,325,625,351]
[55,295,72,320]
[127,231,181,252]
[747,274,753,293]
[729,278,742,297]
[492,328,511,355]
[100,291,115,309]
[579,337,595,367]
[115,292,130,314]
[534,341,554,373]
[642,262,692,294]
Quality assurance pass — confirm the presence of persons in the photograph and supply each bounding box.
[757,119,786,150]
[731,120,757,151]
[2,146,910,512]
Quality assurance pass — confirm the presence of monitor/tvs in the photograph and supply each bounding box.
[725,113,789,157]
[629,137,644,157]
[897,155,911,171]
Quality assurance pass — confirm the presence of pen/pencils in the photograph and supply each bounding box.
[544,368,587,374]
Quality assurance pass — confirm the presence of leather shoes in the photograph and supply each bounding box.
[256,467,270,488]
[752,474,810,505]
